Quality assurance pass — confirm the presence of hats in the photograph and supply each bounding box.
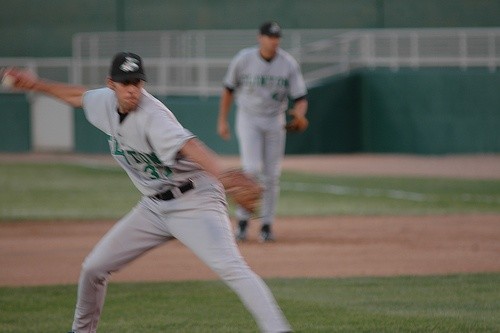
[260,21,282,38]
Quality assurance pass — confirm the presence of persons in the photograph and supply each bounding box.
[218,22,308,243]
[0,53,294,333]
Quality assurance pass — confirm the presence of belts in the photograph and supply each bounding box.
[148,181,194,201]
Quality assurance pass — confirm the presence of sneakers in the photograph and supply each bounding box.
[259,231,273,245]
[237,227,247,243]
[111,52,148,83]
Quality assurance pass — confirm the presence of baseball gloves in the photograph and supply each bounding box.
[285,117,308,132]
[224,168,266,215]
